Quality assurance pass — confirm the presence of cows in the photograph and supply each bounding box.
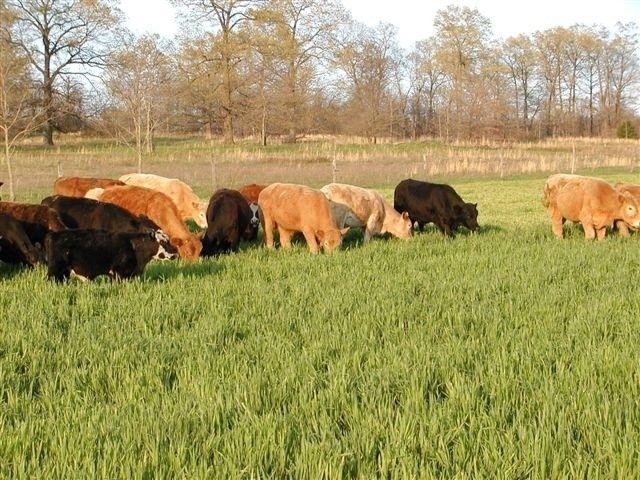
[0,172,416,287]
[394,179,480,240]
[613,181,640,237]
[542,173,640,241]
